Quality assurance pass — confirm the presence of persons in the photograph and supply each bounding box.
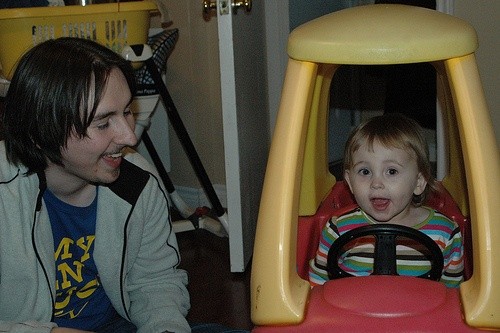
[308,112,464,290]
[0,37,192,333]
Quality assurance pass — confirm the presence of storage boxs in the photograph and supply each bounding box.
[0,1,160,84]
